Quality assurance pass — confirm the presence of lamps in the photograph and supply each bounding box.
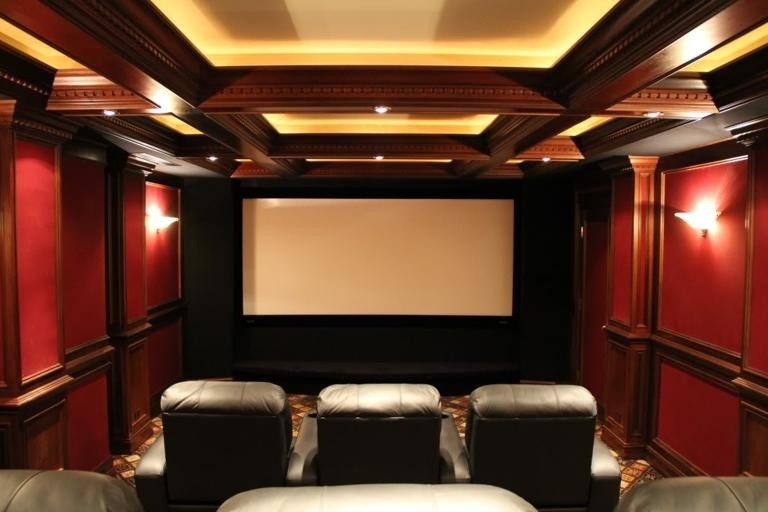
[670,205,729,241]
[141,205,183,235]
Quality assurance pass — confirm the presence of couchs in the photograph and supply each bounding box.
[218,483,540,512]
[463,379,625,512]
[617,476,767,511]
[2,469,143,510]
[284,378,472,486]
[128,376,290,512]
